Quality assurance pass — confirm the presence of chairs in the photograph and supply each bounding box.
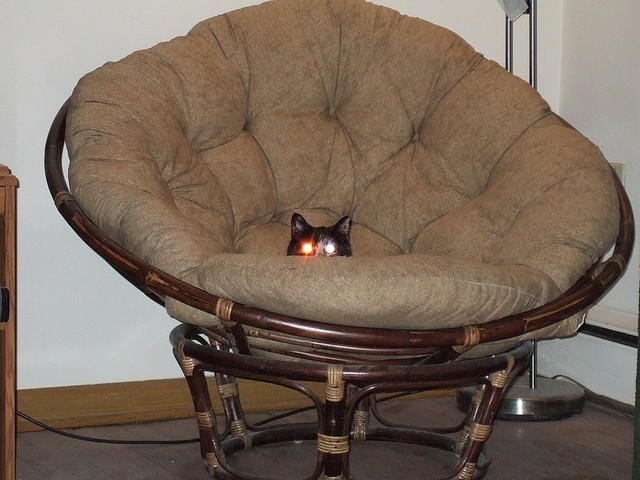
[42,1,635,480]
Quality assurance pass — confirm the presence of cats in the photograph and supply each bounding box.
[287,211,353,255]
